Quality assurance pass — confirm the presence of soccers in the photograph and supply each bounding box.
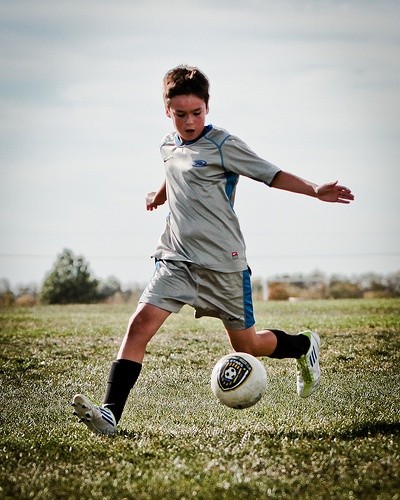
[211,352,268,409]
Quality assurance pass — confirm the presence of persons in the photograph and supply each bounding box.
[71,64,356,438]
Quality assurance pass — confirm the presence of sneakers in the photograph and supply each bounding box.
[71,394,118,437]
[294,330,321,398]
